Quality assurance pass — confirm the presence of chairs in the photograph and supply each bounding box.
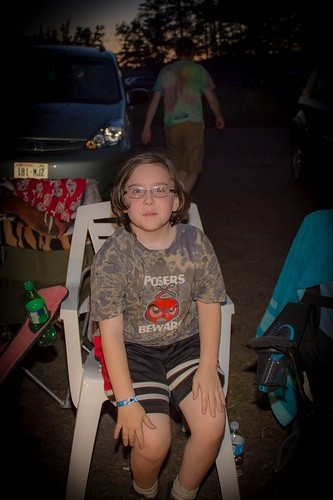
[59,201,239,500]
[246,211,333,500]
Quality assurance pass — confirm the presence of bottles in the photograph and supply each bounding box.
[230,421,245,477]
[24,280,57,346]
[258,353,283,393]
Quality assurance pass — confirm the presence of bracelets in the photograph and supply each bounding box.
[116,395,138,407]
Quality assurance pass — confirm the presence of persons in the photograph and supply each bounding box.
[90,151,227,500]
[141,38,224,194]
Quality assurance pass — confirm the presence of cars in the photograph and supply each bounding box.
[124,76,156,89]
[293,48,332,190]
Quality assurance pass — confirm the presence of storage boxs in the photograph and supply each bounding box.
[0,242,94,284]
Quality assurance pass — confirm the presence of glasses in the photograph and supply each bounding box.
[123,185,177,199]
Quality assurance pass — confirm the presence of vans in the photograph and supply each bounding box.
[0,45,132,203]
